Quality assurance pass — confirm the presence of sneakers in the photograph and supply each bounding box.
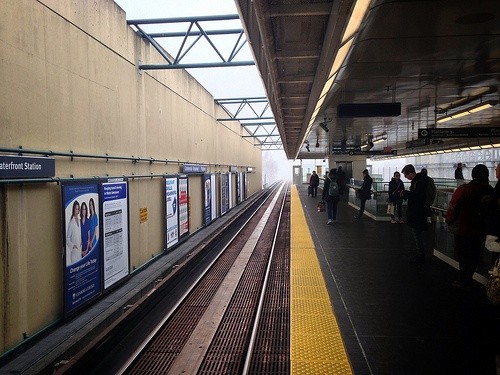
[327,219,336,224]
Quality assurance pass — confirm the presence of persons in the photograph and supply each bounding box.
[310,171,319,198]
[353,169,372,218]
[421,169,437,220]
[388,171,405,223]
[89,198,98,251]
[338,166,347,201]
[445,164,500,292]
[67,200,82,263]
[462,164,469,180]
[400,165,426,262]
[455,162,464,188]
[80,202,91,257]
[322,168,342,225]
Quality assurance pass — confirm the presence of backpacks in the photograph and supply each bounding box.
[329,177,339,197]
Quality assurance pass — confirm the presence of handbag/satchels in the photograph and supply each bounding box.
[316,202,326,212]
[387,205,395,215]
[486,256,500,303]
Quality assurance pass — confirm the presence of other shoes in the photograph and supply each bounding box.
[353,210,364,219]
[406,255,425,264]
[452,280,461,287]
[391,218,397,223]
[399,218,404,223]
